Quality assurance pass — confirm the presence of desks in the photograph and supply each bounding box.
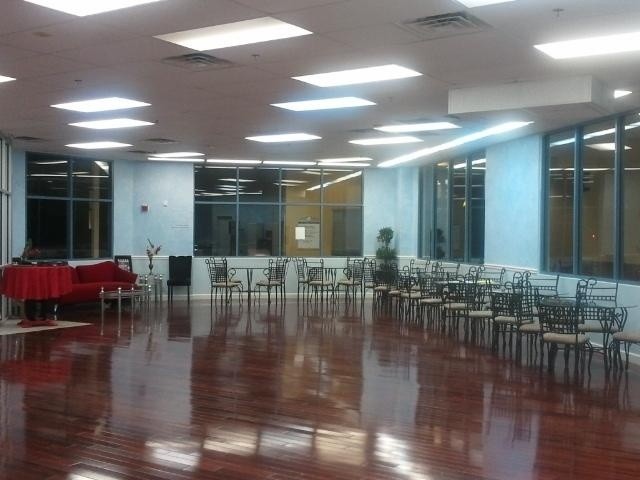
[0,262,73,326]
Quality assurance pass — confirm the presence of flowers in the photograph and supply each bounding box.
[145,238,162,258]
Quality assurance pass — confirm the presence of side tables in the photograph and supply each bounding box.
[138,273,167,303]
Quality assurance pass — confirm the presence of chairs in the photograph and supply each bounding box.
[167,304,192,341]
[115,256,132,273]
[166,256,192,304]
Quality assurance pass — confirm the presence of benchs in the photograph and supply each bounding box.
[46,261,138,315]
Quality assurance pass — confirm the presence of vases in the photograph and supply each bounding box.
[149,258,153,275]
